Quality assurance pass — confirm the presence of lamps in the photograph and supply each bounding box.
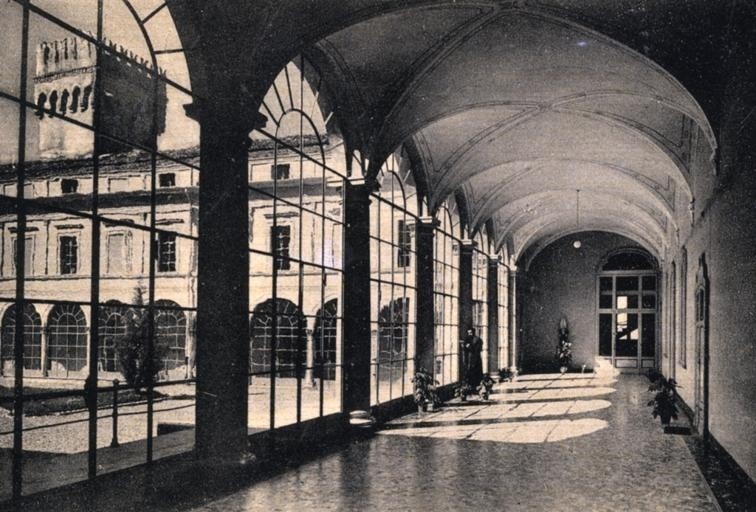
[572,191,581,249]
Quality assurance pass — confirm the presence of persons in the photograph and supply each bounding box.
[460,328,484,388]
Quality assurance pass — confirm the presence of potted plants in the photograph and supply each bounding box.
[410,341,575,412]
[646,376,681,426]
